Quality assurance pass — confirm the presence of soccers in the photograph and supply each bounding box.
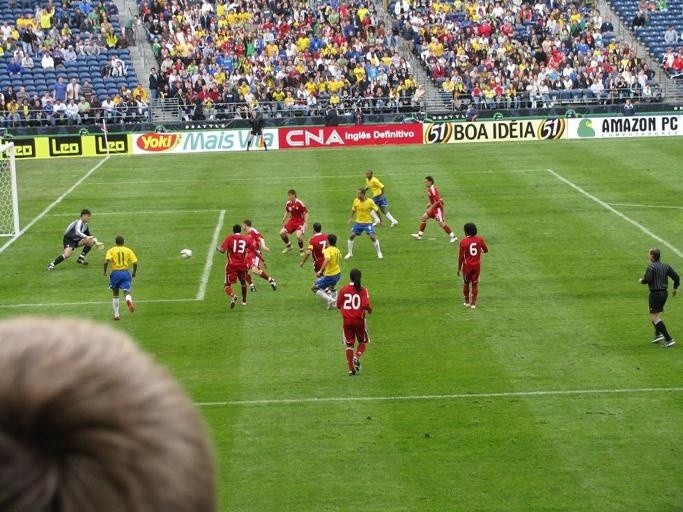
[181,249,192,258]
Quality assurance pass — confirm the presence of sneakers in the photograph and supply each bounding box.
[327,298,336,310]
[650,334,665,343]
[242,301,246,306]
[352,358,360,372]
[270,278,277,290]
[126,299,135,312]
[463,302,471,307]
[391,220,398,227]
[450,237,458,243]
[300,247,304,256]
[411,234,421,240]
[77,257,88,265]
[250,284,256,292]
[373,220,381,226]
[662,339,676,347]
[348,370,355,375]
[470,304,475,309]
[282,246,293,254]
[230,296,237,308]
[344,253,353,259]
[114,314,120,320]
[47,263,54,272]
[378,252,383,259]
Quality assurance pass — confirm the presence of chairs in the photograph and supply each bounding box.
[550,90,593,100]
[514,24,529,41]
[601,0,683,60]
[0,0,140,102]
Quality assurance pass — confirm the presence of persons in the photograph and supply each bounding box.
[139,0,424,126]
[664,25,678,43]
[638,248,680,347]
[103,236,138,320]
[0,0,138,78]
[216,224,265,309]
[242,219,270,252]
[1,314,217,511]
[457,222,488,308]
[300,222,332,295]
[364,170,398,227]
[425,1,662,121]
[244,235,277,291]
[47,209,95,271]
[0,78,150,135]
[411,176,458,243]
[247,111,267,151]
[311,234,343,309]
[662,46,683,78]
[344,188,384,259]
[279,189,309,256]
[336,269,372,375]
